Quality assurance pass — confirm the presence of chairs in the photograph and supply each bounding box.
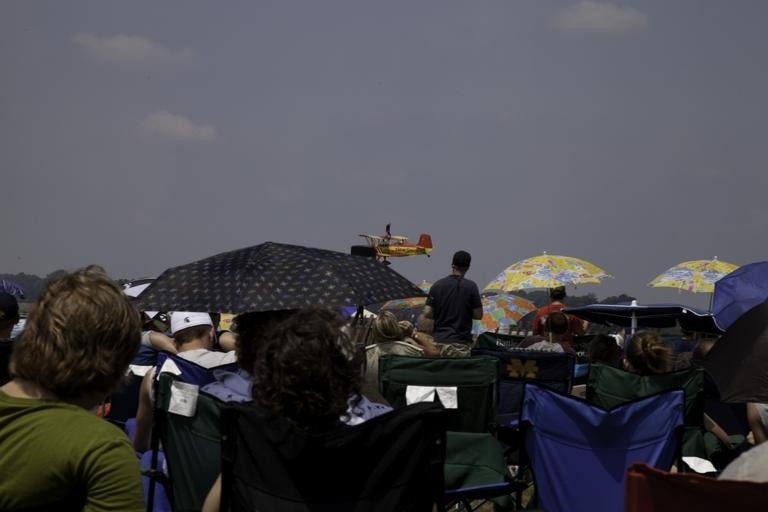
[213,397,450,511]
[585,363,708,473]
[471,347,577,485]
[623,460,766,510]
[520,381,686,510]
[378,354,524,510]
[128,333,262,511]
[358,340,425,408]
[475,332,708,404]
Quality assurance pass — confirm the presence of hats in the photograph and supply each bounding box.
[171,311,218,345]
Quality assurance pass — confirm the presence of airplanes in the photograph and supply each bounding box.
[357,224,433,265]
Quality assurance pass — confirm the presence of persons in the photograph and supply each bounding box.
[0,241,768,511]
